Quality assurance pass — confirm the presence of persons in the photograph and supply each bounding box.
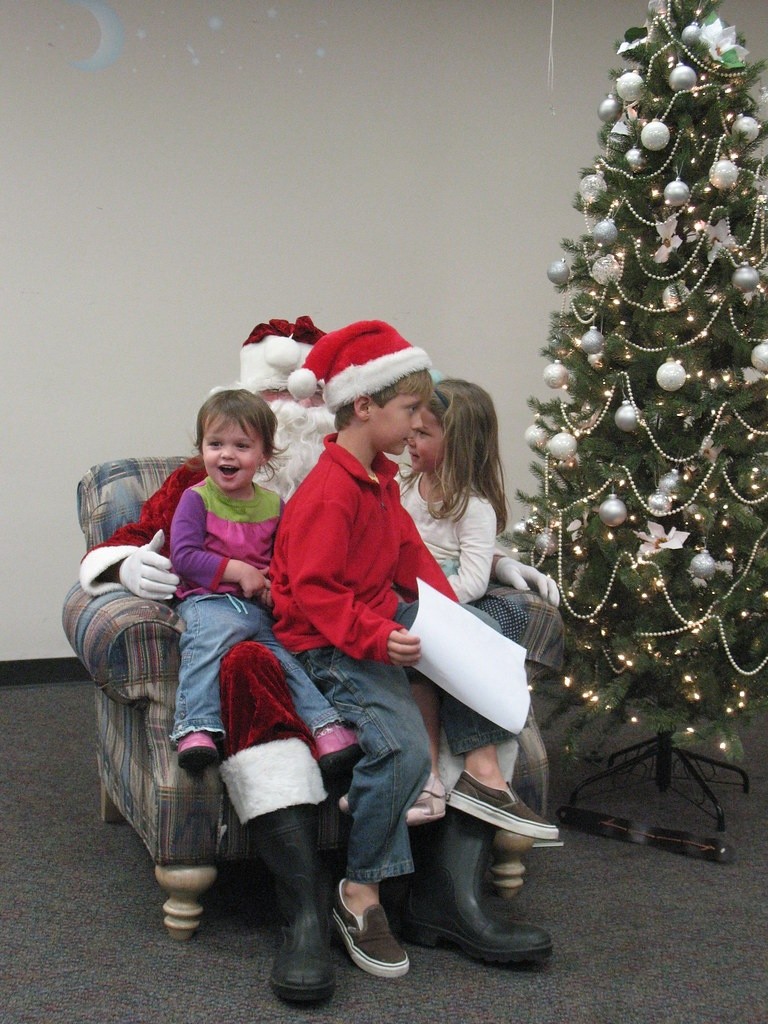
[270,320,560,978]
[167,389,359,773]
[337,378,506,825]
[78,317,552,1002]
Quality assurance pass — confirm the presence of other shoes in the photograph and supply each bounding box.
[177,731,220,770]
[331,878,410,978]
[314,719,364,771]
[339,793,350,813]
[405,772,446,825]
[445,770,560,841]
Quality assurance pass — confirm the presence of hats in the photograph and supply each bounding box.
[240,316,329,393]
[287,320,433,415]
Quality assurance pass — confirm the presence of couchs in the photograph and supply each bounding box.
[60,454,566,939]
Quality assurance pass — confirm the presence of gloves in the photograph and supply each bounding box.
[118,528,180,600]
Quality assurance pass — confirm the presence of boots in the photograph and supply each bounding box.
[397,802,555,964]
[247,817,337,1000]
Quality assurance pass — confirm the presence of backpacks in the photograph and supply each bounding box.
[495,557,560,608]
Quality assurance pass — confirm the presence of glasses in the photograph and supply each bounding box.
[261,386,326,407]
